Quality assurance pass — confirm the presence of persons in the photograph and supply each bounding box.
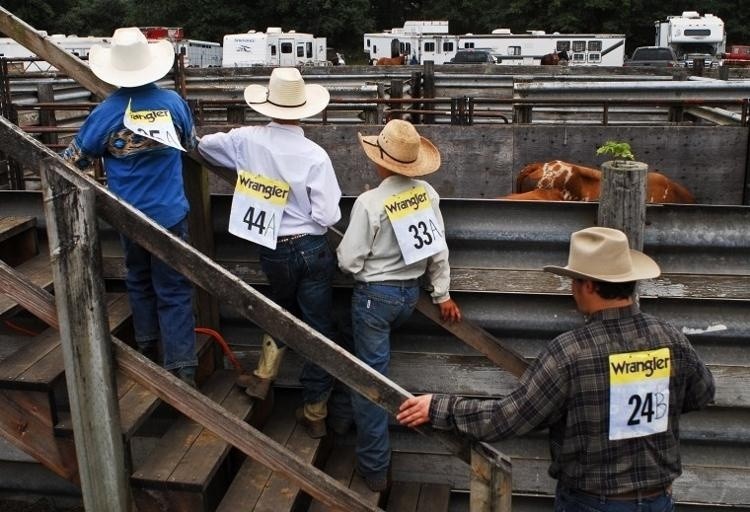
[330,56,339,66]
[335,119,460,486]
[53,26,198,389]
[558,47,569,61]
[395,229,714,510]
[197,66,341,438]
[409,55,418,64]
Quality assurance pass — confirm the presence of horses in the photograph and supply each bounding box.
[376,56,405,65]
[541,49,569,65]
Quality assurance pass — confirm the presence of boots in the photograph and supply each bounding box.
[295,397,327,438]
[236,332,288,400]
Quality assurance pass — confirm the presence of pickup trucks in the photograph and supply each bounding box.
[444,49,495,65]
[622,46,678,68]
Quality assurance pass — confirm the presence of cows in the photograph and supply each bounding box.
[496,187,578,200]
[516,159,694,205]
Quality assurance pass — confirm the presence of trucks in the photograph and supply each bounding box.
[1,31,222,71]
[223,26,327,66]
[655,10,728,67]
[722,46,750,66]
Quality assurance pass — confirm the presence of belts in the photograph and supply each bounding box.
[557,476,674,503]
[359,278,420,289]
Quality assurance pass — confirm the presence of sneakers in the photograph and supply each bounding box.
[174,364,198,390]
[328,415,353,436]
[137,340,158,363]
[354,457,389,492]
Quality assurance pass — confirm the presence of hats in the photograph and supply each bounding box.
[543,226,662,284]
[88,26,176,88]
[358,118,442,178]
[242,67,331,122]
